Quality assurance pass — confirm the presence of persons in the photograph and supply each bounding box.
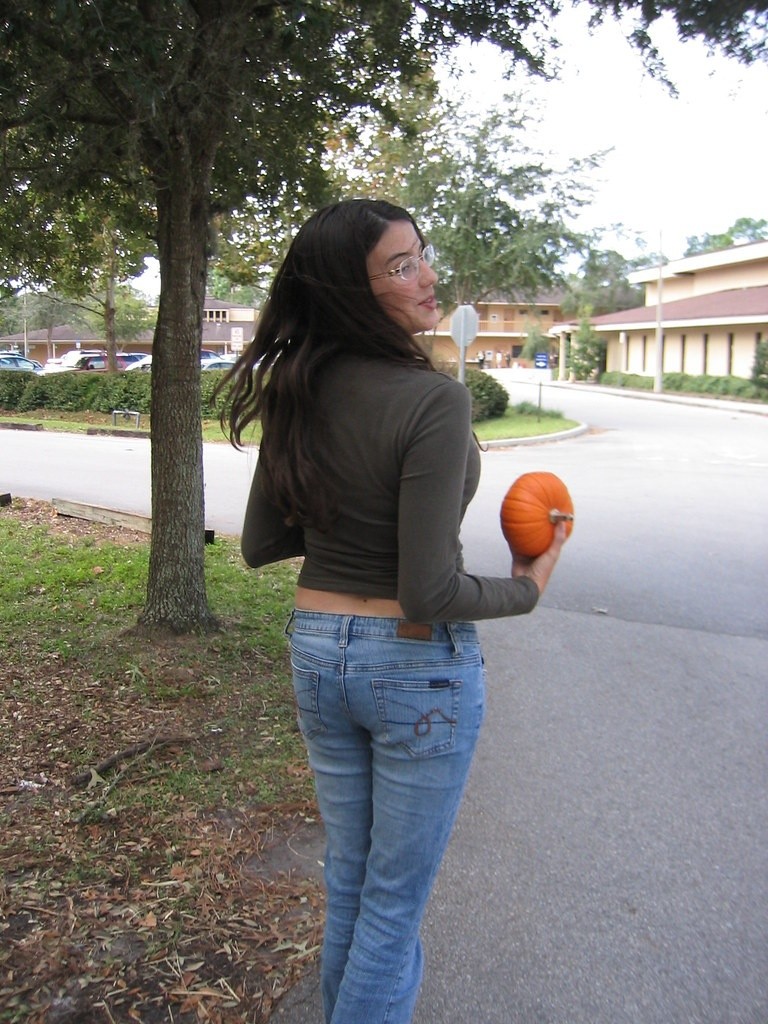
[207,198,567,1024]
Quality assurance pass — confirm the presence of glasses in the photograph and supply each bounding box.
[370,244,435,280]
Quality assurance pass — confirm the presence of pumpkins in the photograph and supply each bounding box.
[500,472,575,558]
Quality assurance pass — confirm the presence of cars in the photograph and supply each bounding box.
[0,349,243,371]
